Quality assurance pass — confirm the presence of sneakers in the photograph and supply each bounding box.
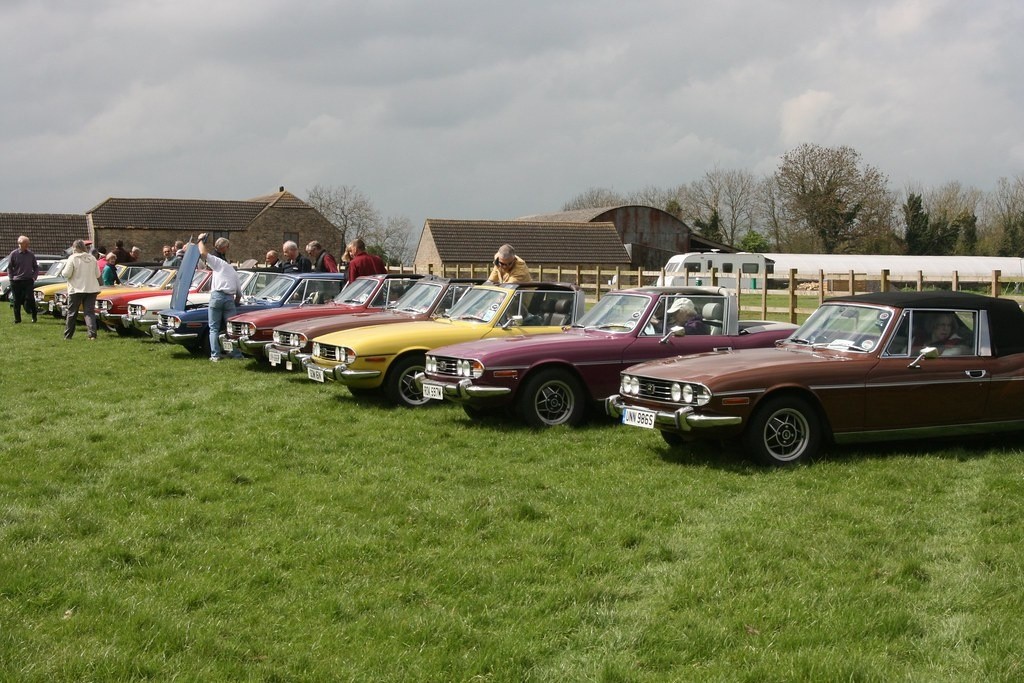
[209,357,220,362]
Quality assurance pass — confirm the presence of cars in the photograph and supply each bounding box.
[0,253,463,372]
[303,281,628,409]
[611,287,1024,473]
[421,285,803,433]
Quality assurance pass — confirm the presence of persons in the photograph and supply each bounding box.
[7,235,39,323]
[207,237,229,269]
[901,313,963,357]
[59,238,140,340]
[266,239,388,285]
[650,297,706,335]
[162,241,187,267]
[196,233,242,362]
[483,243,535,315]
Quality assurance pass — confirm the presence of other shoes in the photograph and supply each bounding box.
[32,320,36,323]
[89,336,96,340]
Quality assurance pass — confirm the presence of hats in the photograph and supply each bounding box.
[83,240,92,245]
[106,252,117,260]
[667,298,694,313]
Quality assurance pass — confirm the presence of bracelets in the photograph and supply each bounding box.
[197,239,203,244]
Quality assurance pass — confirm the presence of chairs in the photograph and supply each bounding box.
[535,298,572,326]
[701,303,723,335]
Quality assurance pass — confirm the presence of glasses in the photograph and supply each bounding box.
[496,261,515,269]
